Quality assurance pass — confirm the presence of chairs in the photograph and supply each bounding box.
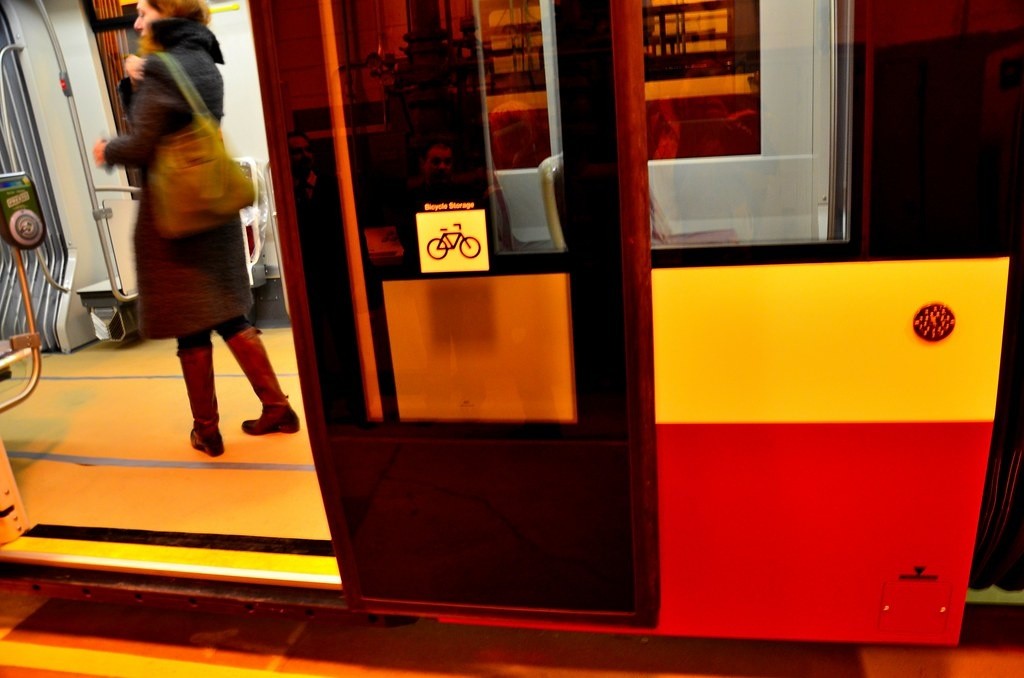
[229,155,269,289]
[491,151,742,254]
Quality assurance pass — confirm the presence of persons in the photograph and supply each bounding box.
[95,0,300,458]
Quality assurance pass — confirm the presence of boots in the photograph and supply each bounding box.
[175,344,224,458]
[226,326,299,436]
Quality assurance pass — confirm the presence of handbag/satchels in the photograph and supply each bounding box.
[146,50,257,238]
